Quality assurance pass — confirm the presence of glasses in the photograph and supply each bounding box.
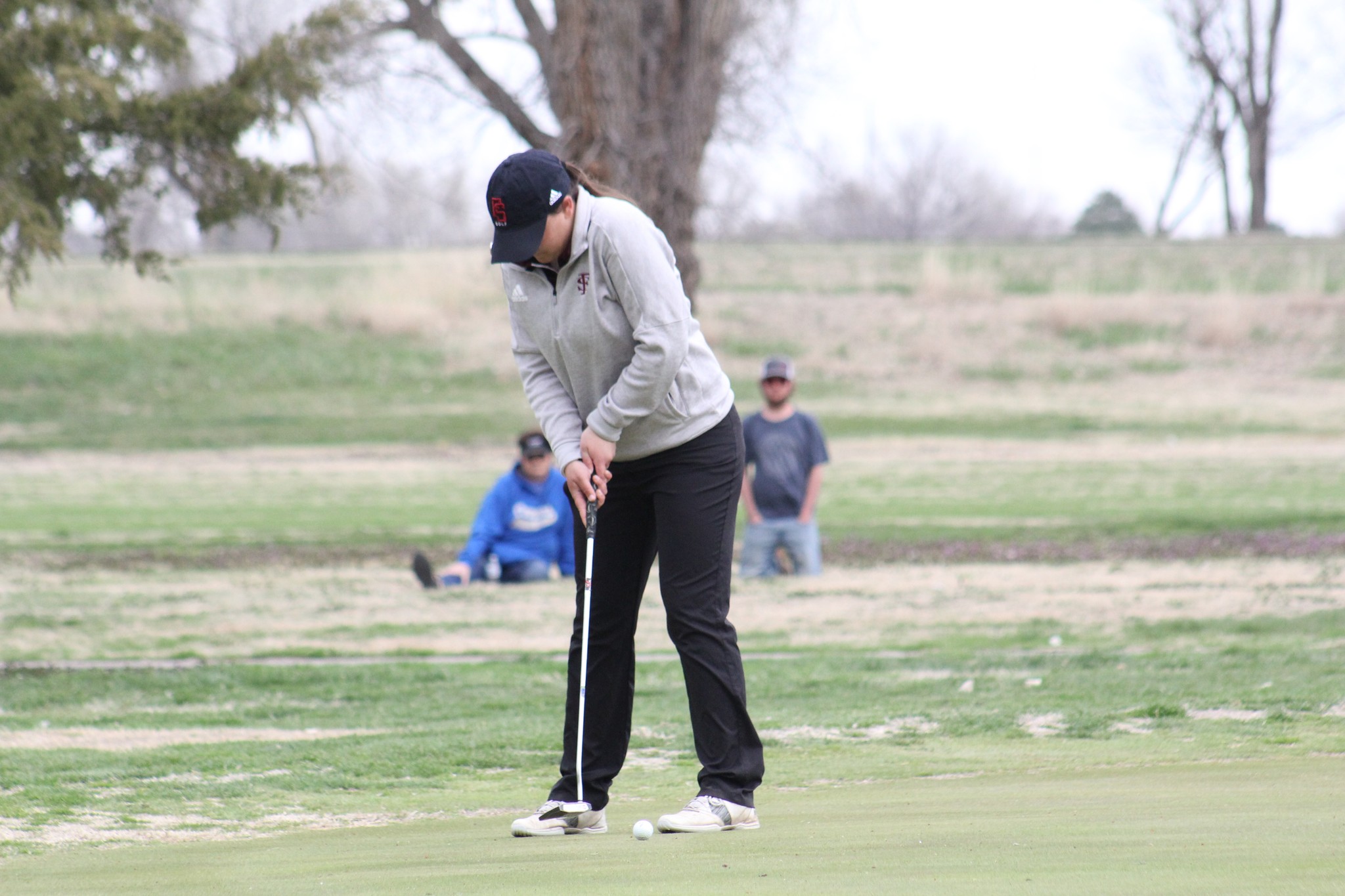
[527,451,546,460]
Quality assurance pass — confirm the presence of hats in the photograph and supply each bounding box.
[761,359,794,382]
[486,150,571,261]
[520,432,552,458]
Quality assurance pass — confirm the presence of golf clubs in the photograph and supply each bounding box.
[559,469,598,813]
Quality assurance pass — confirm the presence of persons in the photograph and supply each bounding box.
[485,148,769,837]
[410,430,579,590]
[740,356,832,584]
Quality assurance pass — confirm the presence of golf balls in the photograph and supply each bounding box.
[633,820,653,840]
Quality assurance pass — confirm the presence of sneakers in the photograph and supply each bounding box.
[657,796,760,832]
[511,800,607,836]
